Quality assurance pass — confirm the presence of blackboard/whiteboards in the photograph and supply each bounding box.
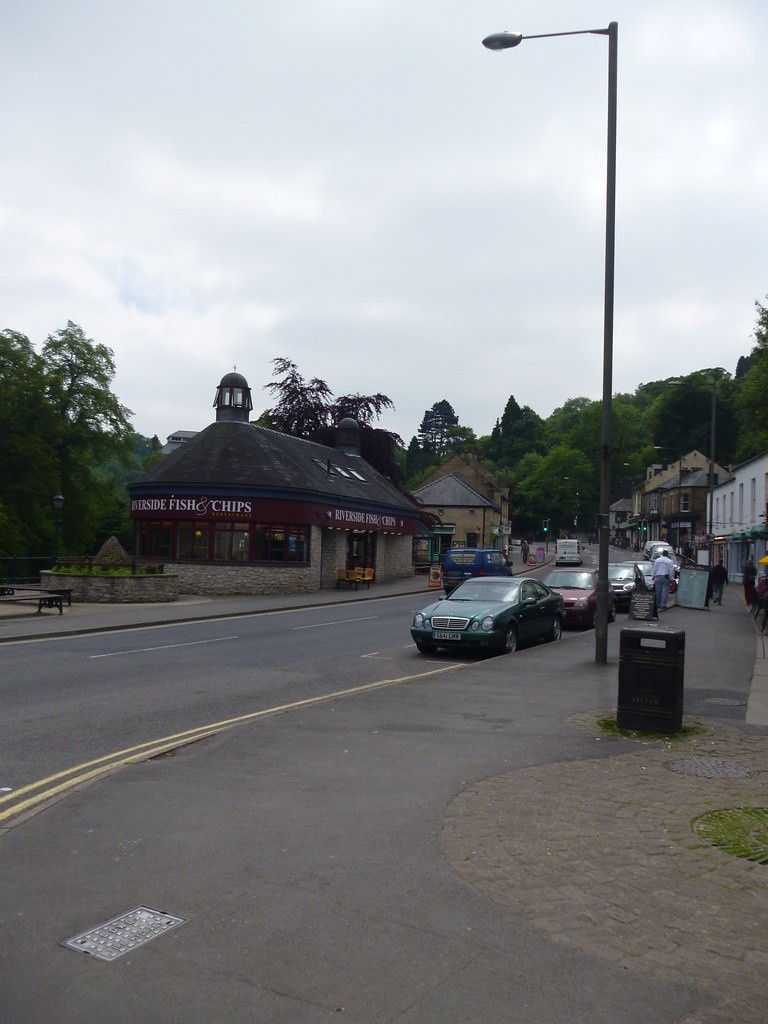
[629,590,658,620]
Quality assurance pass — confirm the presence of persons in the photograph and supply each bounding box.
[743,558,757,607]
[710,558,729,605]
[520,540,531,564]
[653,550,674,609]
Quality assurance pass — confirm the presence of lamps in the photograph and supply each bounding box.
[320,511,332,518]
[400,520,404,527]
[438,507,443,511]
[470,507,474,511]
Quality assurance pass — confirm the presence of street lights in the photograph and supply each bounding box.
[654,446,682,547]
[51,488,65,569]
[481,21,620,666]
[563,477,580,539]
[667,380,716,533]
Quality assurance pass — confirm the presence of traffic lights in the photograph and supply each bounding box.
[643,520,648,531]
[638,520,642,530]
[542,520,548,533]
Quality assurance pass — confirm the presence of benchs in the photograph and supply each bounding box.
[0,587,75,606]
[0,594,64,616]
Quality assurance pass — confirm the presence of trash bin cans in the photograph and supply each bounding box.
[617,625,686,734]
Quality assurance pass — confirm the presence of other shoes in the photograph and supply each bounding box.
[713,595,719,603]
[660,607,667,610]
[657,606,660,610]
[718,602,722,604]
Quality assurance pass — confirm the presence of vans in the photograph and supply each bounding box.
[442,548,514,595]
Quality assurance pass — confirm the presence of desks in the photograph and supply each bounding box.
[343,570,367,591]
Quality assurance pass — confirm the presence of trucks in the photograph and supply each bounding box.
[556,537,584,567]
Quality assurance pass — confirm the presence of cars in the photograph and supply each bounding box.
[609,563,646,612]
[624,560,654,591]
[542,566,617,632]
[644,540,682,580]
[410,576,567,659]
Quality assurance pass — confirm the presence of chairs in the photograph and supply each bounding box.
[337,567,375,590]
